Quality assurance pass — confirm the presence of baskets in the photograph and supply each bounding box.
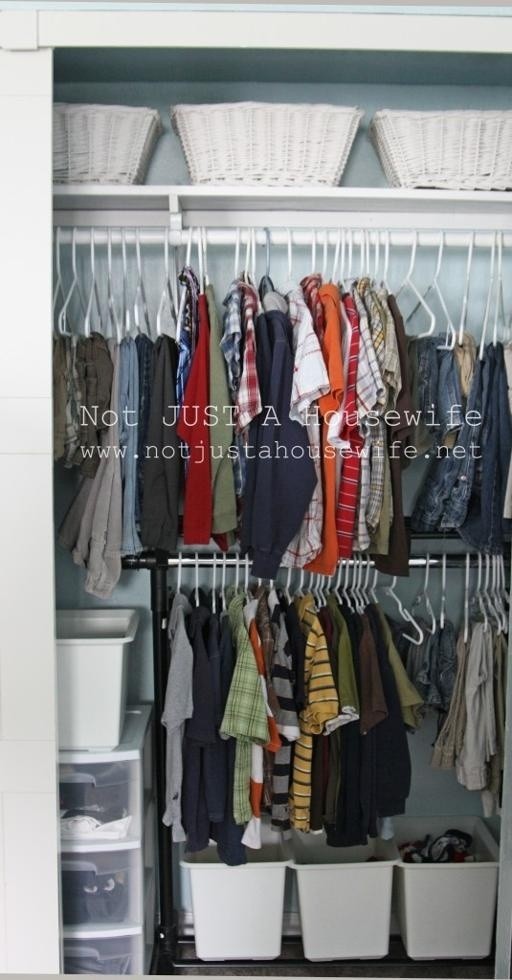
[366,109,512,191]
[168,101,365,188]
[53,102,164,185]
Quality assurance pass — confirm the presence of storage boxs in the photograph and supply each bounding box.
[178,841,293,964]
[288,841,400,963]
[396,816,499,961]
[55,609,142,751]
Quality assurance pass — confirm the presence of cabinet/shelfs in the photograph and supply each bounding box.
[57,703,159,975]
[52,44,512,979]
